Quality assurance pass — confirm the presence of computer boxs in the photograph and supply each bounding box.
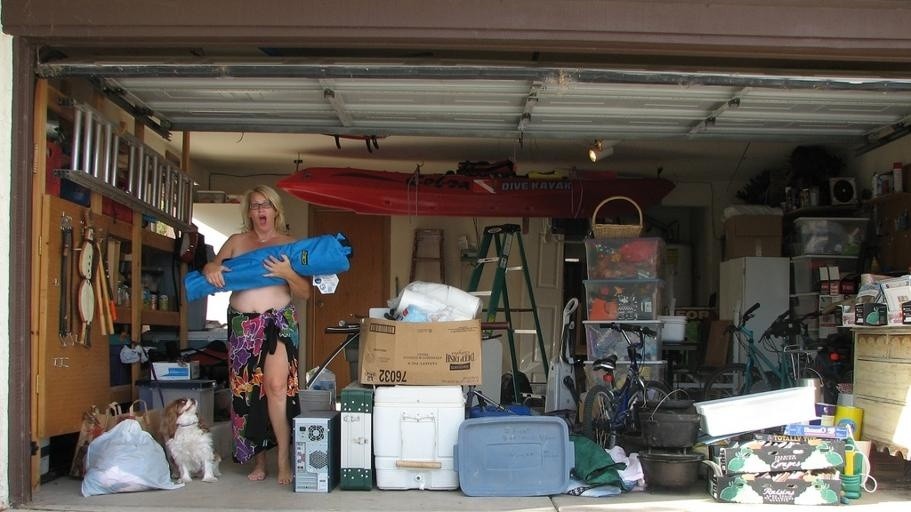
[292,410,340,495]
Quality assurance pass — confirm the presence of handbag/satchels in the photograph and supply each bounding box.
[69,402,123,479]
[107,399,160,438]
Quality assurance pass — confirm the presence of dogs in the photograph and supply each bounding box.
[164,397,222,484]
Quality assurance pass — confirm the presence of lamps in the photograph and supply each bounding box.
[589,138,613,164]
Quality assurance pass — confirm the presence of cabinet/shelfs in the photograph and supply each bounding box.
[852,329,911,461]
[28,78,188,490]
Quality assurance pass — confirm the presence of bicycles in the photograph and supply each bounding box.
[724,305,841,410]
[585,323,675,449]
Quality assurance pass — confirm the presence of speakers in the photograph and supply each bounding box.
[828,175,856,206]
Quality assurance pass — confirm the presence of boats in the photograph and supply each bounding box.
[277,166,677,219]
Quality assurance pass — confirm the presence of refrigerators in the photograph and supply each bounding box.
[719,257,789,375]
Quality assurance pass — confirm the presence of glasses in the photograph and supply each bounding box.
[249,202,273,209]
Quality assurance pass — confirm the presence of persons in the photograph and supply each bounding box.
[202,182,312,486]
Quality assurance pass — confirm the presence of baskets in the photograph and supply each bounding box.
[592,196,643,238]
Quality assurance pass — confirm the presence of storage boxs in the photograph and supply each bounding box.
[791,294,818,328]
[135,380,214,429]
[692,387,815,437]
[793,256,858,293]
[585,238,666,280]
[793,217,868,255]
[582,320,664,361]
[453,417,574,498]
[710,444,846,473]
[583,280,663,320]
[584,361,665,400]
[724,216,782,259]
[373,387,464,490]
[708,478,842,505]
[358,317,481,385]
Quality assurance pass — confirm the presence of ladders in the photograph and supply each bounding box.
[52,97,199,234]
[467,223,548,403]
